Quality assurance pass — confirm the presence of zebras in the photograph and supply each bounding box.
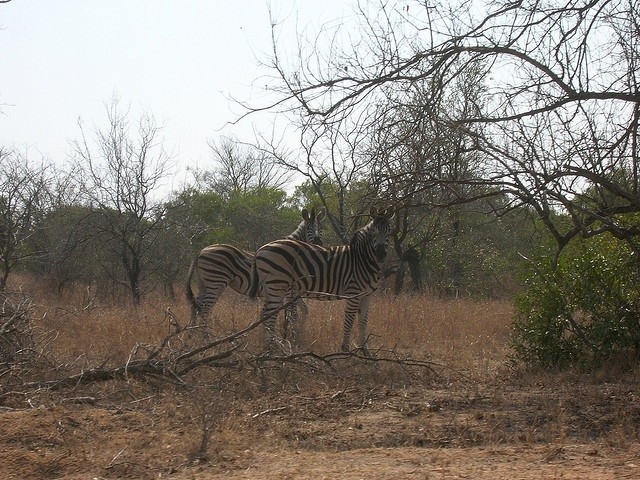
[247,204,396,364]
[183,205,326,347]
